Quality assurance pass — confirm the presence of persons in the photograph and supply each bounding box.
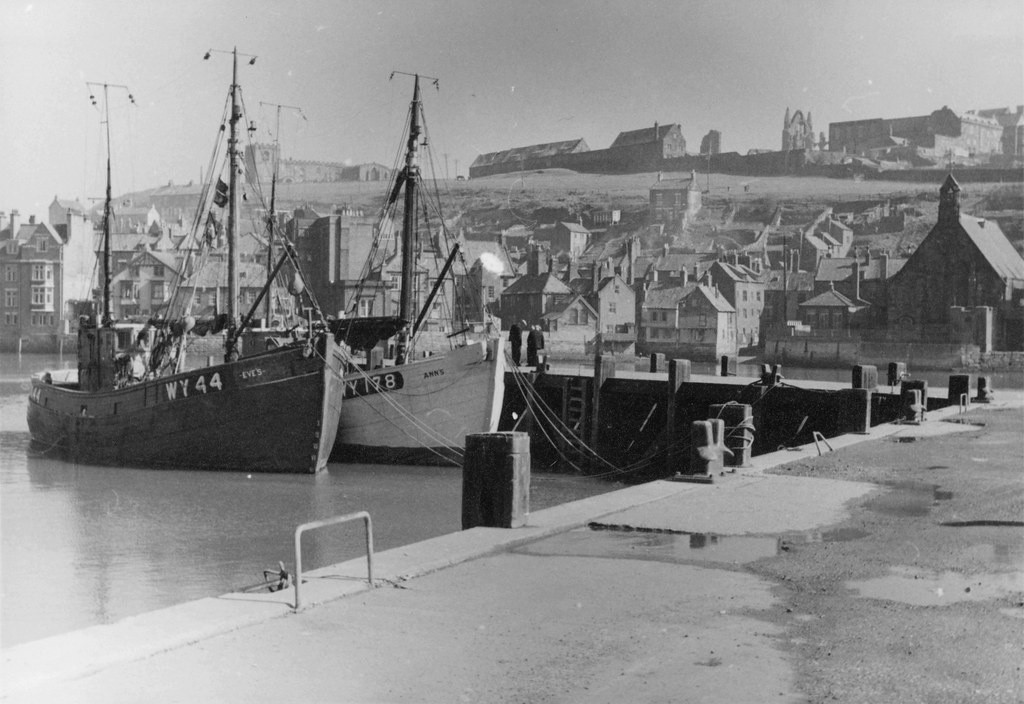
[509,319,545,366]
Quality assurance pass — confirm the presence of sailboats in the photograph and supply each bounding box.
[23,45,352,476]
[239,67,509,467]
